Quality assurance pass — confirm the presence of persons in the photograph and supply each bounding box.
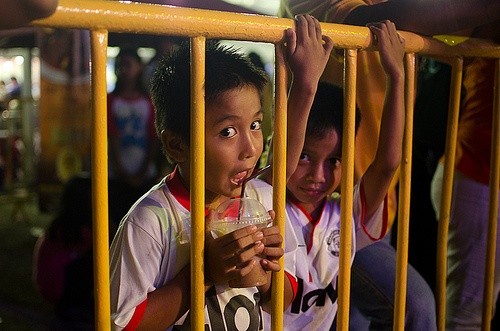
[259,15,406,331]
[107,41,297,331]
[272,0,500,331]
[0,0,59,41]
[0,77,20,110]
[108,51,168,198]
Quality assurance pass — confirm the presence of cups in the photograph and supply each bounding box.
[208,197,272,289]
